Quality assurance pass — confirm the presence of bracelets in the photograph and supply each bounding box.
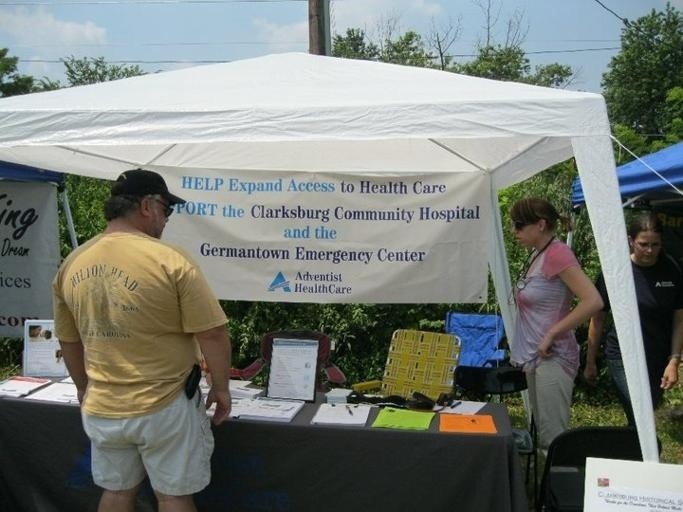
[669,354,681,358]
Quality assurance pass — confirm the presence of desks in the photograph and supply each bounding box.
[0,368,529,512]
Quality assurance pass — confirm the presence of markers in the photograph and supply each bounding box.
[346,405,354,415]
[451,401,462,408]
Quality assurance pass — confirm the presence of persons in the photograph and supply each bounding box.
[50,169,232,512]
[584,211,683,457]
[29,326,52,341]
[510,197,604,461]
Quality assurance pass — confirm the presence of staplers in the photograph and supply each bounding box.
[408,392,434,410]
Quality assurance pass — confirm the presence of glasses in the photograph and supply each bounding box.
[633,239,662,250]
[514,220,535,230]
[150,196,174,217]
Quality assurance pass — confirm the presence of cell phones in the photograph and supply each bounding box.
[184,363,203,400]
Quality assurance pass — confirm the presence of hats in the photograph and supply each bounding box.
[110,167,186,205]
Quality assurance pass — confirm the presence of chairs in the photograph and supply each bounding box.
[352,328,461,404]
[541,426,663,512]
[240,330,346,392]
[446,310,506,402]
